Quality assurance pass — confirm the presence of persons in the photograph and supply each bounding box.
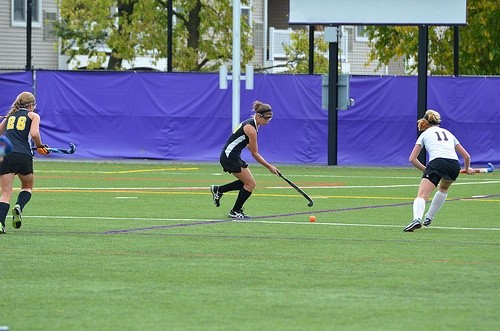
[404,110,476,232]
[211,100,279,219]
[0,92,51,234]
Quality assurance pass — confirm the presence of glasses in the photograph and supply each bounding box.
[263,116,271,121]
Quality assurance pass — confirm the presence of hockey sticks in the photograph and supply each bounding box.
[31,142,77,155]
[277,170,314,207]
[459,162,495,174]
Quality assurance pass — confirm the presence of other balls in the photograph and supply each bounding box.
[309,215,316,222]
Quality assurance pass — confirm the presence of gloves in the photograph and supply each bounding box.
[37,144,51,155]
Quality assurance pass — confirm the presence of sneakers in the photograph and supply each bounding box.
[229,210,250,219]
[211,185,223,208]
[404,219,421,232]
[12,204,21,229]
[423,219,431,226]
[0,222,6,234]
